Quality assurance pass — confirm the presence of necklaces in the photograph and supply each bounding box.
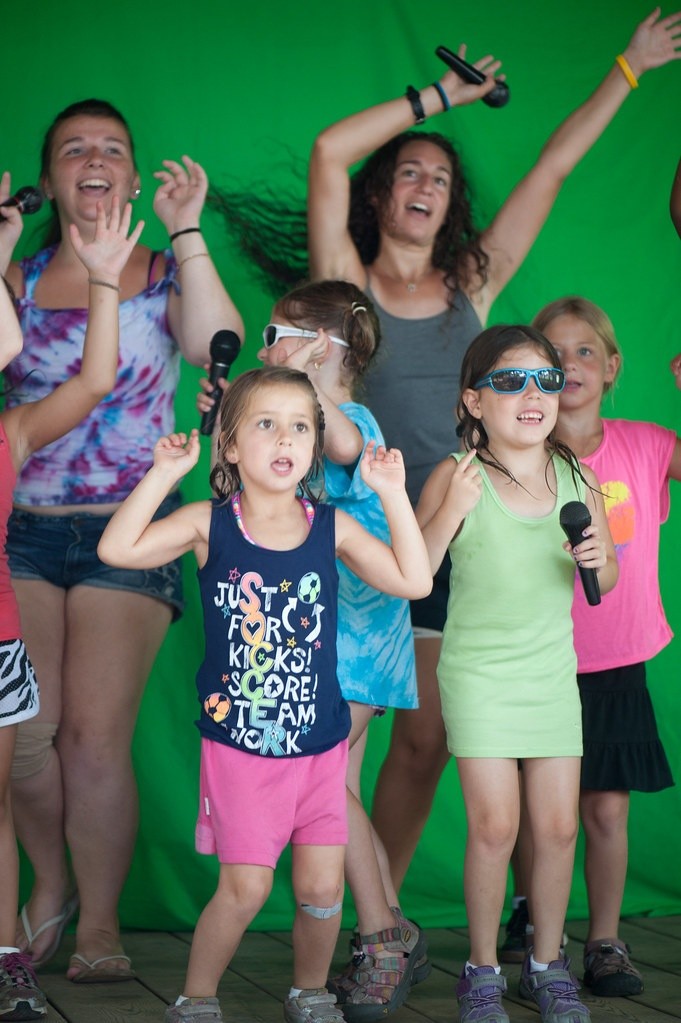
[408,283,417,292]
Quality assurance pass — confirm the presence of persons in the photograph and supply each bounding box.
[0,98,245,1023]
[531,296,681,995]
[97,366,433,1023]
[225,7,681,961]
[196,279,419,1023]
[414,325,619,1023]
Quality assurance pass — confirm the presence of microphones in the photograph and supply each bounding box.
[0,186,42,222]
[559,501,602,607]
[435,45,510,108]
[199,329,241,436]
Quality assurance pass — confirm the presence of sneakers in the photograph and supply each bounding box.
[518,946,592,1023]
[454,965,510,1023]
[329,905,427,1023]
[0,951,48,1021]
[582,938,644,997]
[325,952,431,1004]
[283,988,347,1023]
[499,899,528,963]
[166,996,223,1023]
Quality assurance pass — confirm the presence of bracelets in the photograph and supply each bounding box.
[615,54,639,89]
[178,253,208,268]
[87,278,119,291]
[170,227,201,243]
[405,86,425,126]
[432,82,451,111]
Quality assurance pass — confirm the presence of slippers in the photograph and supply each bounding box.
[21,893,80,970]
[68,953,137,983]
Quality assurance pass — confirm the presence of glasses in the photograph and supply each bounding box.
[263,324,352,348]
[471,368,566,393]
